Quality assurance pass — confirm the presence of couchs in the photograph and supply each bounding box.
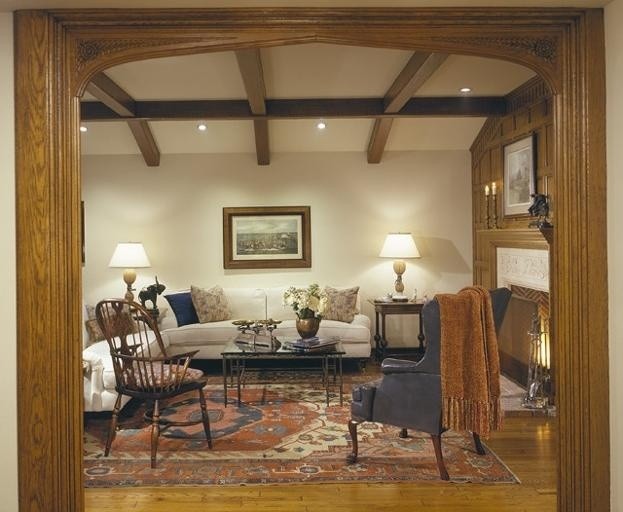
[81,282,371,415]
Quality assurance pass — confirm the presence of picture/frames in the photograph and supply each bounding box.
[498,130,539,221]
[221,205,312,271]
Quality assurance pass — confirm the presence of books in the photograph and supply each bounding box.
[288,336,341,349]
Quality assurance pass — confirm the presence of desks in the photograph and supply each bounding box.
[369,299,425,365]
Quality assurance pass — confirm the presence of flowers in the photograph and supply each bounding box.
[280,280,333,318]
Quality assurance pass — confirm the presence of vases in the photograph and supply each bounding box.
[294,316,319,337]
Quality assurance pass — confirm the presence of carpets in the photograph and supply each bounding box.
[81,365,526,487]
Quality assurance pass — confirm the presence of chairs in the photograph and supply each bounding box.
[93,298,214,472]
[344,289,517,484]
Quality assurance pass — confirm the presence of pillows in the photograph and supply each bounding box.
[165,291,198,328]
[316,284,360,322]
[190,282,231,323]
[85,290,178,340]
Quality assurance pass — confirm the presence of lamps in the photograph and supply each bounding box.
[106,241,151,312]
[378,232,421,303]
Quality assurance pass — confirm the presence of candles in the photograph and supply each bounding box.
[491,181,497,195]
[484,184,488,195]
